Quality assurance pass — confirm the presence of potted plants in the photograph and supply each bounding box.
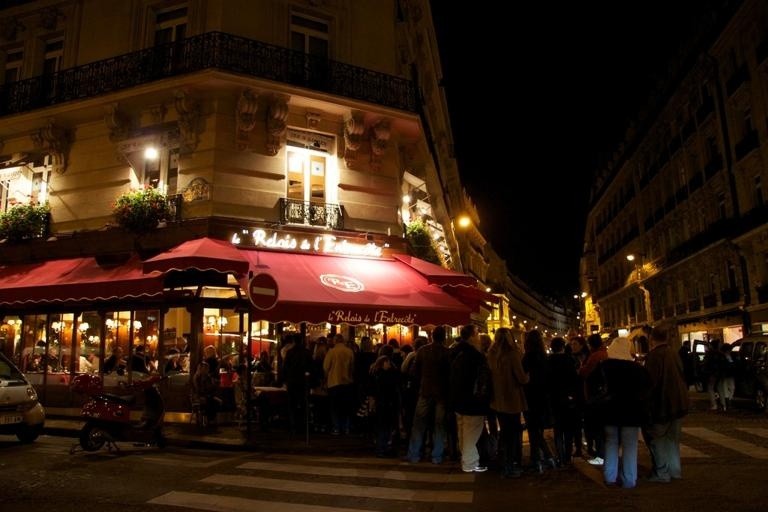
[0,203,49,245]
[408,218,430,257]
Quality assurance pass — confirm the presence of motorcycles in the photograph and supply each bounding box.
[69,372,170,451]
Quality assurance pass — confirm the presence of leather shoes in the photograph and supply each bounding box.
[313,424,449,464]
[495,450,582,478]
[644,470,670,483]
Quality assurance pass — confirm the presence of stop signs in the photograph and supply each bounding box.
[247,272,280,311]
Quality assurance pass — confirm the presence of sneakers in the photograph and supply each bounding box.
[462,465,488,472]
[587,456,604,466]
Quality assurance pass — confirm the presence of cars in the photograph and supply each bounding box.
[0,351,46,442]
[692,336,767,410]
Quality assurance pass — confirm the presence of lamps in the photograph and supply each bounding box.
[456,209,473,233]
[140,142,162,165]
[7,311,268,349]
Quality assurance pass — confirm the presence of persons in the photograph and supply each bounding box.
[274,321,738,489]
[24,336,279,439]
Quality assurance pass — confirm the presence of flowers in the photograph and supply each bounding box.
[110,184,175,224]
